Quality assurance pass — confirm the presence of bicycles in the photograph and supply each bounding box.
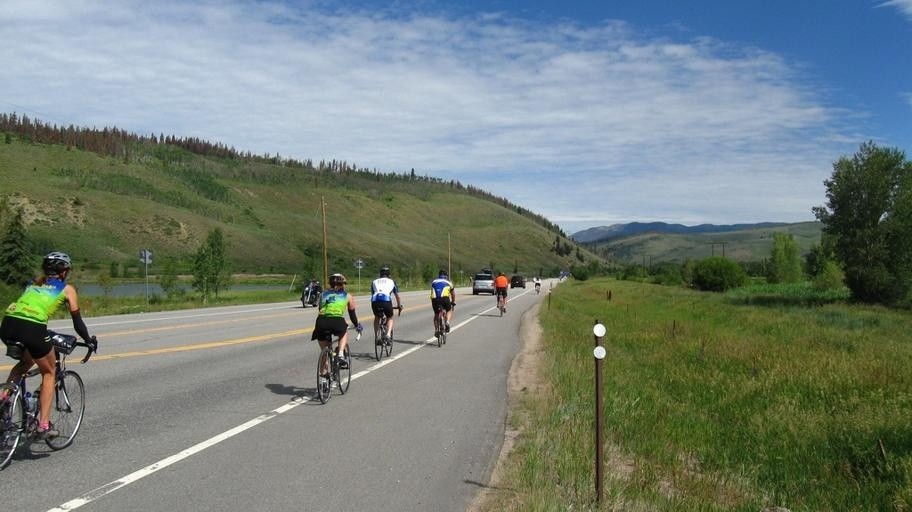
[433,303,457,348]
[0,332,99,472]
[535,285,540,295]
[317,321,363,404]
[497,291,506,317]
[373,305,403,361]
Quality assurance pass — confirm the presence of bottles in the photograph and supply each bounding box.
[25,392,36,412]
[32,384,42,403]
[331,351,337,362]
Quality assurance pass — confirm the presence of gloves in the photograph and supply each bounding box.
[84,334,99,353]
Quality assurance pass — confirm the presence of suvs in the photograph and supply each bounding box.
[472,273,496,296]
[509,275,527,290]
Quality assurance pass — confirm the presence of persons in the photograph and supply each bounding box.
[430,268,456,338]
[300,277,319,303]
[311,274,362,379]
[535,277,541,289]
[493,271,507,313]
[371,267,402,344]
[1,251,97,440]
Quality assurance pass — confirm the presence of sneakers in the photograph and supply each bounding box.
[319,360,328,376]
[0,387,11,401]
[435,329,440,338]
[445,320,451,332]
[334,355,347,366]
[375,334,391,346]
[36,421,60,438]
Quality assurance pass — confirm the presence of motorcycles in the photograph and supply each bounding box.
[300,277,322,308]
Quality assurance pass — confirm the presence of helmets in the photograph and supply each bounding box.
[380,268,390,278]
[499,271,506,276]
[329,273,346,288]
[439,269,448,276]
[42,251,72,275]
